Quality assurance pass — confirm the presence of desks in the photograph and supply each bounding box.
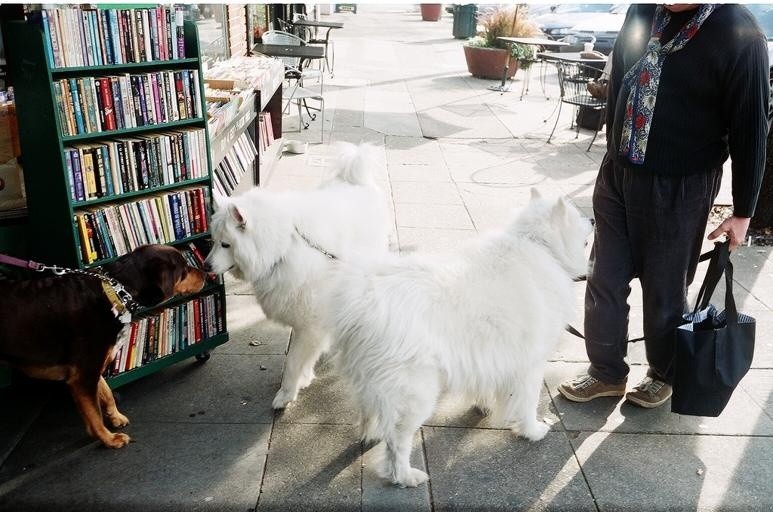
[293,20,343,84]
[536,51,609,128]
[248,43,323,131]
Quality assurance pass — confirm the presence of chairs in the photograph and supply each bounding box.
[261,31,312,115]
[546,61,611,152]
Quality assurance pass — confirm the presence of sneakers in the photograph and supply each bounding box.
[558,375,628,402]
[626,377,673,409]
[587,79,609,98]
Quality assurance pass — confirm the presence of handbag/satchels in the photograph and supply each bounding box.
[666,304,756,417]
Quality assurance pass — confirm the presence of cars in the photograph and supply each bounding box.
[476,4,772,130]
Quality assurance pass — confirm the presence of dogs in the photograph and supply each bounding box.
[201,142,390,413]
[331,188,594,489]
[1,244,208,447]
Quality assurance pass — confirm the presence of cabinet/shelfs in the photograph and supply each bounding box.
[21,3,230,407]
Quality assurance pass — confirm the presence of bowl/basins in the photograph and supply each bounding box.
[285,139,309,154]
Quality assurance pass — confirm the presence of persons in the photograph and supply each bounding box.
[554,4,770,408]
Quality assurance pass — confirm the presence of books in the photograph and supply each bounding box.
[41,5,185,69]
[64,129,208,203]
[180,243,213,288]
[108,293,227,378]
[199,2,281,199]
[52,70,203,139]
[77,187,209,264]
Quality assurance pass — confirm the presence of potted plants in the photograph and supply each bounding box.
[461,3,542,79]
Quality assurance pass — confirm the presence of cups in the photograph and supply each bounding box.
[584,42,595,52]
